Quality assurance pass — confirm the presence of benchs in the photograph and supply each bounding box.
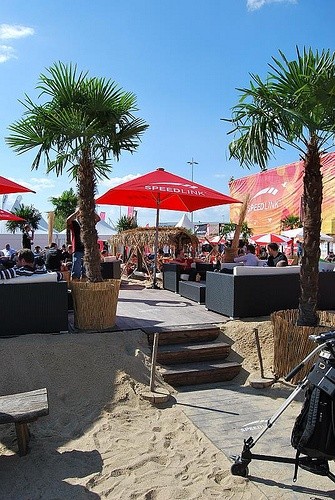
[0,388,49,456]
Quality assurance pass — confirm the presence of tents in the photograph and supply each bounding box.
[0,197,195,234]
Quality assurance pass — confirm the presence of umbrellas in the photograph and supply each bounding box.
[95,168,244,289]
[0,209,27,222]
[175,214,333,245]
[0,176,36,195]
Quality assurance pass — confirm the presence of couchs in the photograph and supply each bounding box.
[0,271,71,338]
[163,261,335,320]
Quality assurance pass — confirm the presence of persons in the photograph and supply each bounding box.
[0,242,335,281]
[22,225,35,250]
[66,207,100,280]
